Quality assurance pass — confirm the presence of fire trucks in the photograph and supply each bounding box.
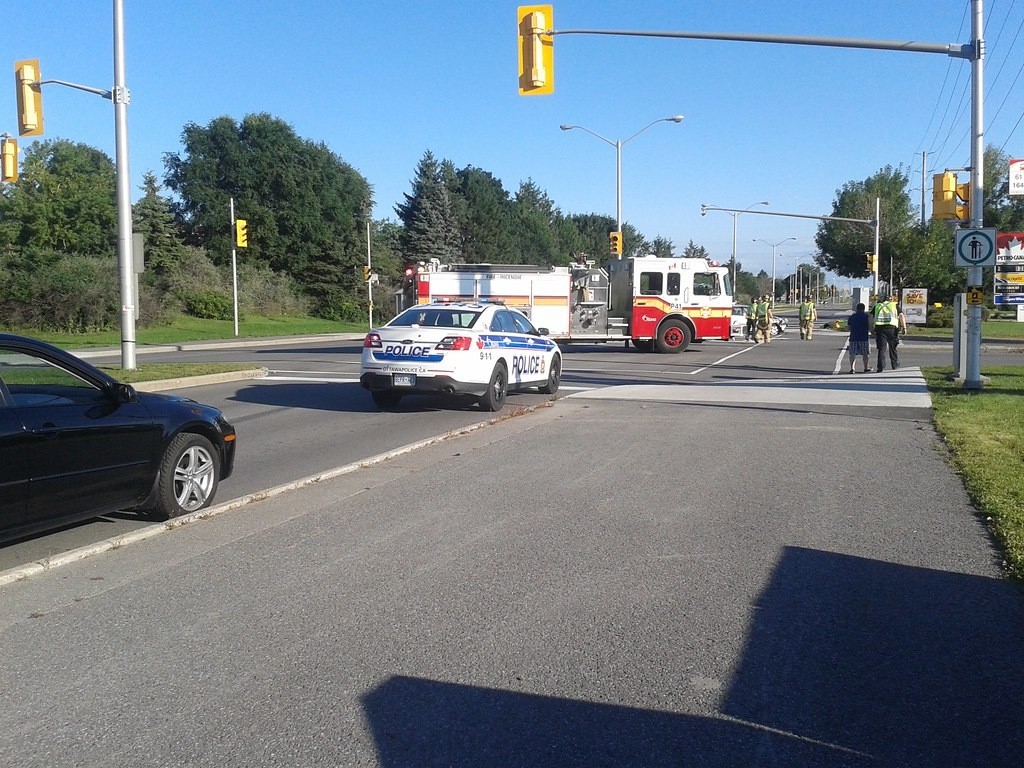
[396,254,734,355]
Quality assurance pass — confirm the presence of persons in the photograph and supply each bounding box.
[799,295,818,340]
[746,297,758,341]
[869,295,907,373]
[753,294,773,343]
[847,303,873,374]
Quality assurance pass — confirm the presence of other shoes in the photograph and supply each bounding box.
[864,367,873,372]
[849,370,855,373]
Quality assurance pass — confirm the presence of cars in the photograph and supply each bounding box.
[0,332,236,545]
[732,304,787,337]
[359,295,563,413]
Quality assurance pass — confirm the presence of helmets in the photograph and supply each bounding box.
[762,295,769,300]
[884,295,892,301]
[807,295,812,301]
[751,297,758,302]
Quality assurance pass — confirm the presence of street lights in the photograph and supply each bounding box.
[752,237,797,310]
[701,201,770,302]
[559,115,685,261]
[780,253,813,306]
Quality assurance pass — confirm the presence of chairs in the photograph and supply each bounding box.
[437,313,454,325]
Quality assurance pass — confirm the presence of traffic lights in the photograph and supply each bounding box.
[867,255,876,272]
[364,266,372,280]
[954,184,969,219]
[609,231,622,255]
[236,219,248,248]
